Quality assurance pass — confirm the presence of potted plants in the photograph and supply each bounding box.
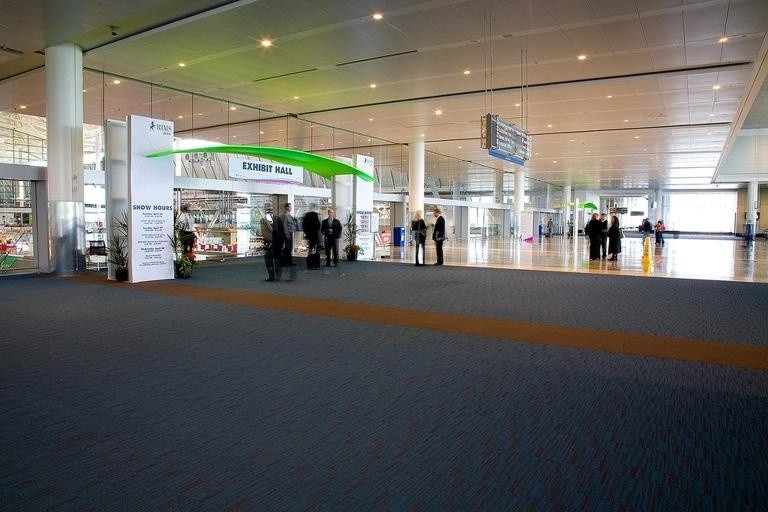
[343,211,361,261]
[166,210,193,278]
[106,232,128,281]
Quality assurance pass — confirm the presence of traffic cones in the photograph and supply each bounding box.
[640,236,652,257]
[640,256,651,277]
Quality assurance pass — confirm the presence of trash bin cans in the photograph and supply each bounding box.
[393,226,405,247]
[539,225,544,235]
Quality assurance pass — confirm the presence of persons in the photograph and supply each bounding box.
[587,213,603,261]
[641,218,665,246]
[258,203,286,281]
[321,206,343,267]
[546,217,554,238]
[173,202,199,255]
[432,208,445,267]
[410,209,429,266]
[599,214,607,260]
[607,215,622,261]
[302,202,322,270]
[567,218,574,239]
[280,202,298,270]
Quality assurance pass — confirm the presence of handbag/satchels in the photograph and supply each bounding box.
[618,229,625,238]
[417,220,427,238]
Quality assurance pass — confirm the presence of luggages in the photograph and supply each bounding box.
[306,242,320,270]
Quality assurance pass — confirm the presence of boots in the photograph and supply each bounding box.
[608,255,617,260]
